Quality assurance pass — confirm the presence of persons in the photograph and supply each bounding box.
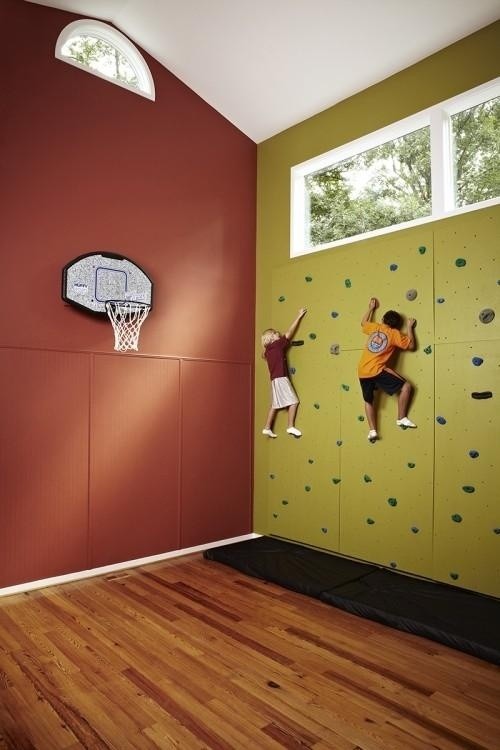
[260,306,309,439]
[358,297,418,441]
